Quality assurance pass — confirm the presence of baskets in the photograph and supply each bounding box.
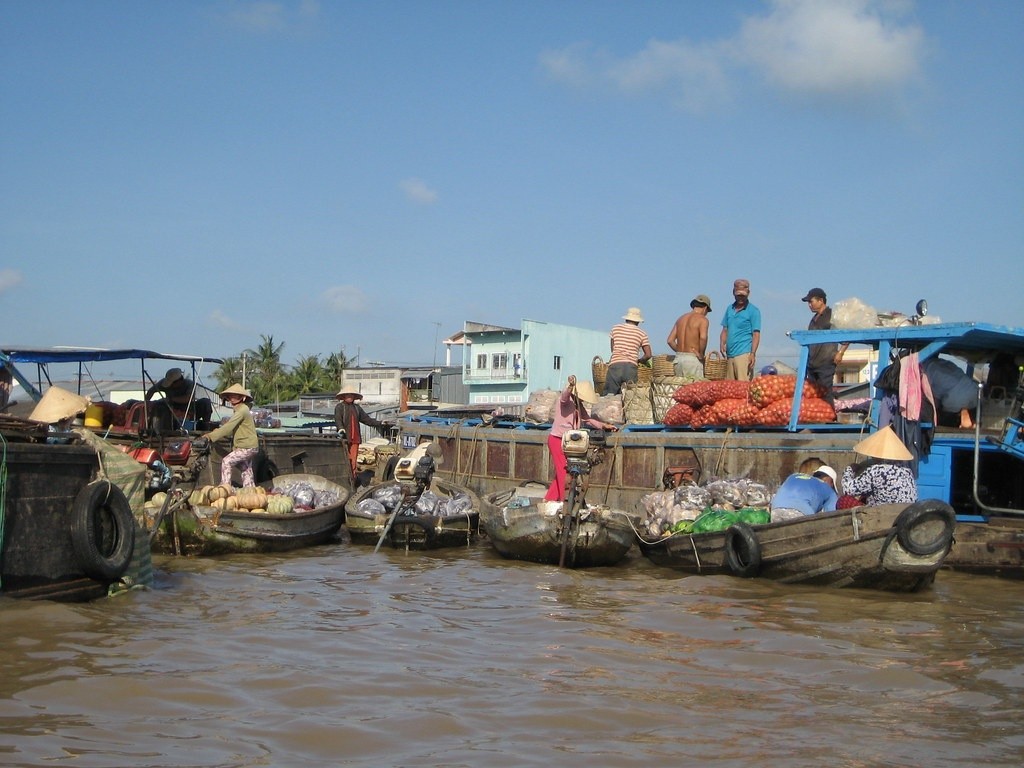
[592,355,609,394]
[704,350,728,380]
[638,365,653,387]
[652,354,676,376]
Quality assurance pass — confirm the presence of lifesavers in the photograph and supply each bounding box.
[253,458,279,484]
[897,498,956,555]
[383,456,399,482]
[385,517,435,550]
[72,480,135,583]
[725,523,759,577]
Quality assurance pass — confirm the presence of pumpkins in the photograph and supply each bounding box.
[145,483,295,515]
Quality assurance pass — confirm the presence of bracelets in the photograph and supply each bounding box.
[750,352,755,356]
[838,351,844,355]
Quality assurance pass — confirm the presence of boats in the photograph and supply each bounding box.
[343,454,481,551]
[637,464,958,593]
[0,343,355,597]
[395,318,1024,579]
[143,469,347,555]
[475,430,641,571]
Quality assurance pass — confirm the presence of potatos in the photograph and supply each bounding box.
[661,372,836,426]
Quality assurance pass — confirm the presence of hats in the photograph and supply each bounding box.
[694,295,712,312]
[734,279,749,296]
[622,308,644,322]
[571,380,600,404]
[29,386,92,424]
[853,426,914,460]
[802,288,827,302]
[812,465,839,493]
[219,383,253,403]
[161,368,181,388]
[336,383,363,400]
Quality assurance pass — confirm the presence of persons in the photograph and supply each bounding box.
[800,288,850,422]
[28,386,92,443]
[145,368,213,430]
[602,308,652,395]
[770,425,919,523]
[201,383,259,489]
[543,375,617,502]
[334,385,389,477]
[667,294,712,379]
[921,355,979,428]
[720,279,761,381]
